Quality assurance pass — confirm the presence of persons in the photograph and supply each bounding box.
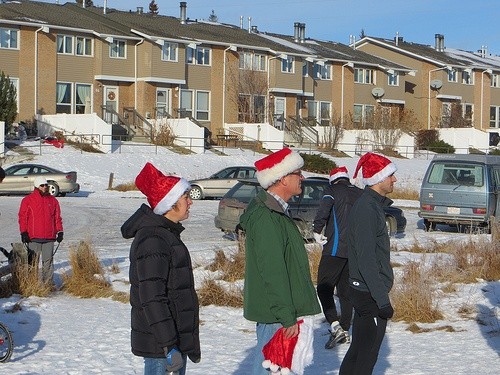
[312,166,357,350]
[239,149,323,375]
[338,153,398,375]
[18,176,64,289]
[120,161,202,375]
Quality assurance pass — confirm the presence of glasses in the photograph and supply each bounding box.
[40,184,48,187]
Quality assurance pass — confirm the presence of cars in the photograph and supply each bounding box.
[214,177,407,240]
[0,163,79,197]
[188,165,259,200]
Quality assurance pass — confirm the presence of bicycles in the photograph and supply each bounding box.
[0,321,14,362]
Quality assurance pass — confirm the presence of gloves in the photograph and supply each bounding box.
[21,232,30,242]
[163,344,184,372]
[56,232,64,243]
[314,232,327,245]
[379,306,394,319]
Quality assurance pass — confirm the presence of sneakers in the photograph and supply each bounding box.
[325,327,345,348]
[337,331,350,343]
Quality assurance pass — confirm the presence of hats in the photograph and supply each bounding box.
[34,176,48,188]
[262,316,313,374]
[135,162,190,215]
[254,147,304,189]
[351,151,398,186]
[329,167,350,185]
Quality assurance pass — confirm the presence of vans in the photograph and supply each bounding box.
[418,153,500,232]
